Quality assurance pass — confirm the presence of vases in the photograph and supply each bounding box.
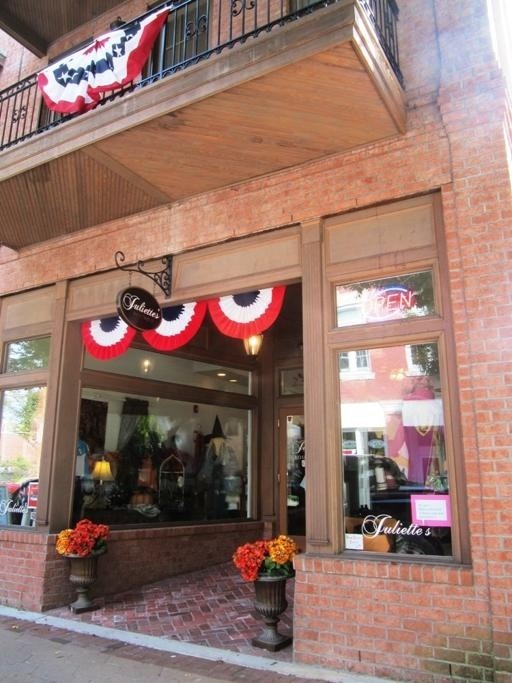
[61,551,105,614]
[228,533,295,583]
[53,518,110,559]
[247,568,294,648]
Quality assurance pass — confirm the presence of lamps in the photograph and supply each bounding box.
[241,329,267,357]
[89,454,118,510]
